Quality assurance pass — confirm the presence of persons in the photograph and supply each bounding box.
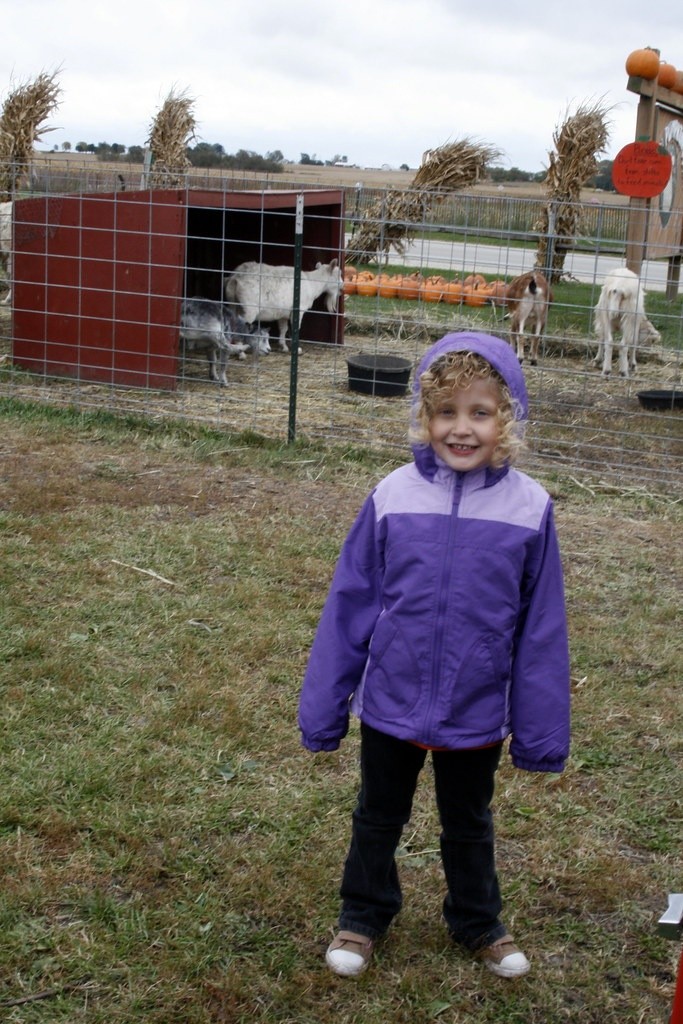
[299,333,570,977]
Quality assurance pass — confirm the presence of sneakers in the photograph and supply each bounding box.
[325,925,375,975]
[479,936,530,979]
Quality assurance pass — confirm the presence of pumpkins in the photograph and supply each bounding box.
[342,264,512,307]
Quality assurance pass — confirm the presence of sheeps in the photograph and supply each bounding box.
[592,267,662,382]
[505,271,554,367]
[179,258,346,390]
[1,240,12,307]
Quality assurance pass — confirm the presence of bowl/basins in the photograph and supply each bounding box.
[346,355,414,396]
[638,390,683,411]
